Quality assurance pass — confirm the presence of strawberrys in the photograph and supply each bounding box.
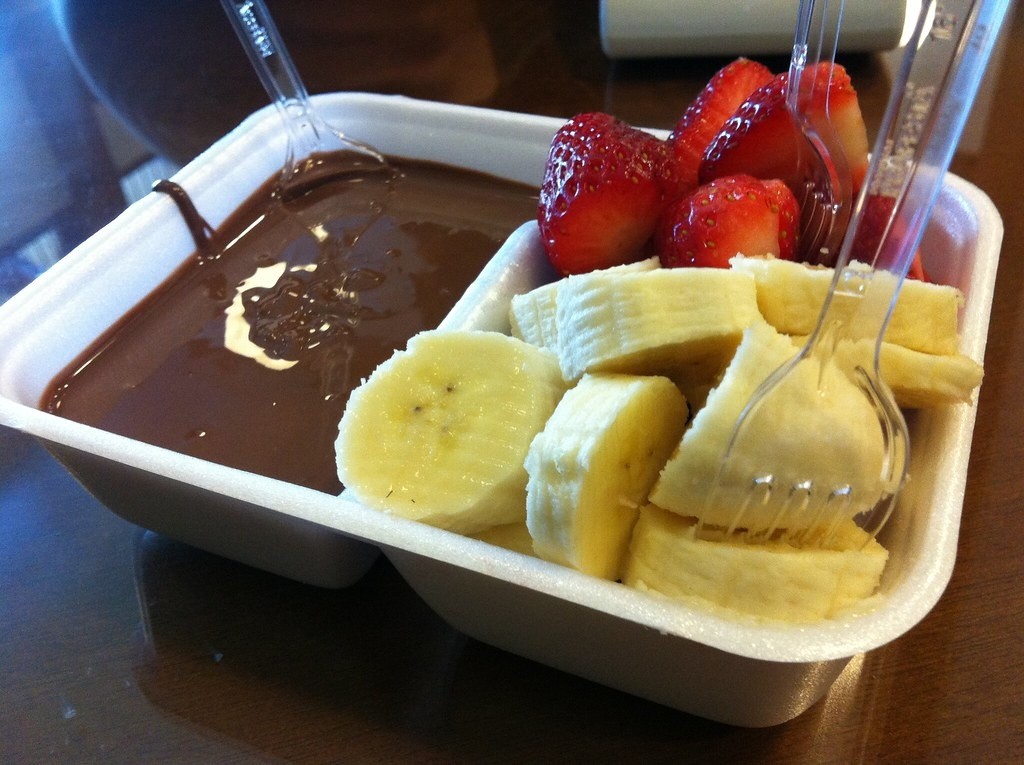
[535,57,925,278]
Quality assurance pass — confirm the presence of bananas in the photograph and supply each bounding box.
[332,255,986,623]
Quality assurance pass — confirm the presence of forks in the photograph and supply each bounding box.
[690,2,1023,544]
